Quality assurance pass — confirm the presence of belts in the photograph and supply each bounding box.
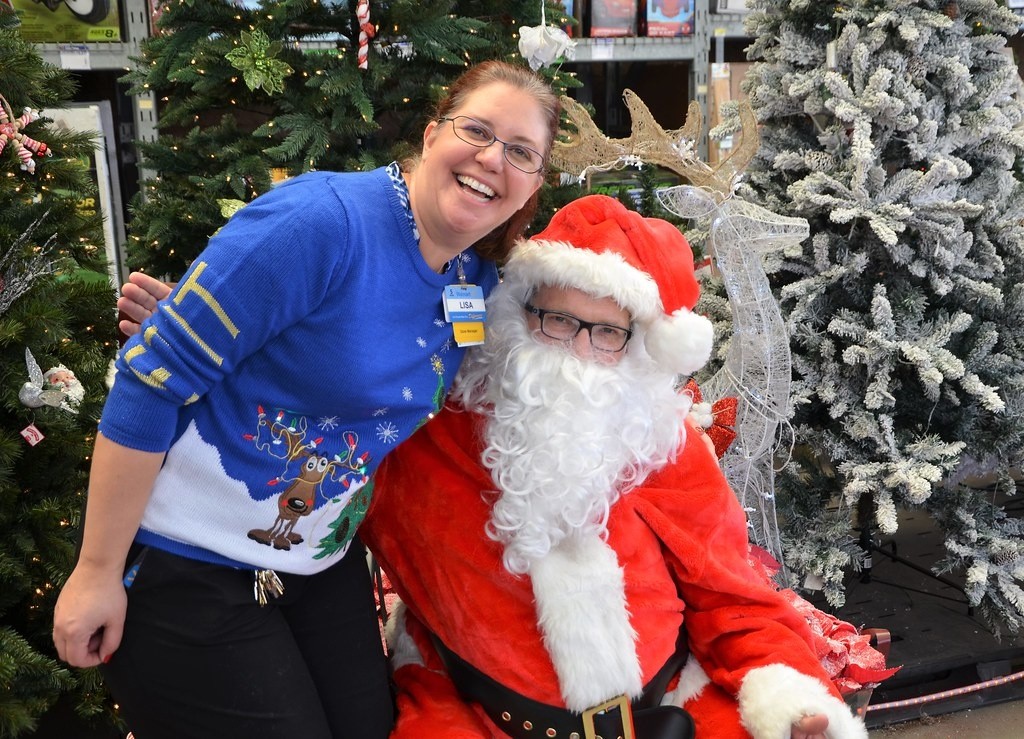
[420,622,698,739]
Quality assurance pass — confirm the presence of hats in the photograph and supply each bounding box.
[506,194,712,376]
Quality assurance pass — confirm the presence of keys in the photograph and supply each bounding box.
[253,570,285,606]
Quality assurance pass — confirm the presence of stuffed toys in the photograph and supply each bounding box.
[42,362,86,415]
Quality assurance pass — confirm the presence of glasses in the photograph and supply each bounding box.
[440,114,548,176]
[526,301,632,352]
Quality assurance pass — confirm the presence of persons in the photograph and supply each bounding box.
[114,193,866,739]
[50,58,562,739]
